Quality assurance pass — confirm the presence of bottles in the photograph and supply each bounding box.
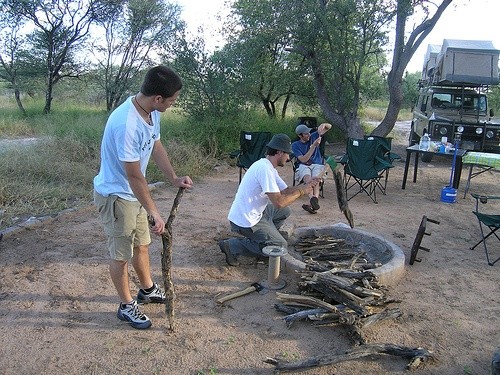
[419,133,431,152]
[440,144,446,153]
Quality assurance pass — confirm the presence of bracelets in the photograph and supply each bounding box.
[293,188,304,199]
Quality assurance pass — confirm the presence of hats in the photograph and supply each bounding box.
[266,133,294,154]
[295,125,312,135]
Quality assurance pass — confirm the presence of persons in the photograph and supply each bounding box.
[218,133,321,266]
[93,66,192,329]
[290,123,333,214]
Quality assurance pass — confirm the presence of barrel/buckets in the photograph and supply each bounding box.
[440,187,457,203]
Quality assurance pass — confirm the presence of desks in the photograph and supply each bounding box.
[403,143,467,190]
[462,151,500,200]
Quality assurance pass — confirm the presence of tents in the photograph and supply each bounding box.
[422,39,500,85]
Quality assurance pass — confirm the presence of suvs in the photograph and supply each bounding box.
[408,87,500,163]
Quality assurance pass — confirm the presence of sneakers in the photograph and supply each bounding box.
[137,282,166,303]
[218,240,239,266]
[117,299,152,329]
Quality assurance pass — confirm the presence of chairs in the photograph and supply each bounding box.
[340,134,402,203]
[288,134,336,198]
[468,191,500,266]
[230,131,271,184]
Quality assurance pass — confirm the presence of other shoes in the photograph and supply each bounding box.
[302,205,317,214]
[310,197,320,210]
